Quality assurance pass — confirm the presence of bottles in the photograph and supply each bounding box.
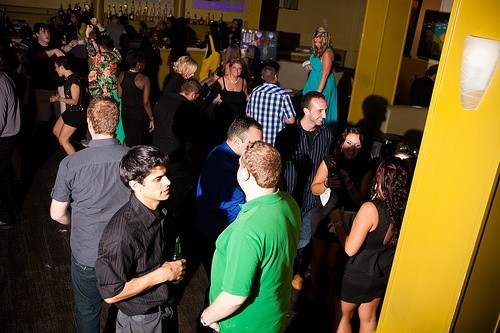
[58,0,90,15]
[108,0,174,23]
[186,12,224,26]
[171,237,184,283]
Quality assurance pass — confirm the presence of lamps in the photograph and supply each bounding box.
[461,35,500,112]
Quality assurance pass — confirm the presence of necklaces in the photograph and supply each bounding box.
[229,78,239,83]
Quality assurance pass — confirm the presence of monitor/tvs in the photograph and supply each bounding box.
[416,9,450,62]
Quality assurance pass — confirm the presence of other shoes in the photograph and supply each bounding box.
[291,273,303,290]
[0,220,12,229]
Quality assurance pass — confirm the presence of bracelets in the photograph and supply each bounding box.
[323,181,328,188]
[334,221,342,227]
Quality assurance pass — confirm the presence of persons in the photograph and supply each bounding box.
[0,14,416,333]
[411,64,438,108]
